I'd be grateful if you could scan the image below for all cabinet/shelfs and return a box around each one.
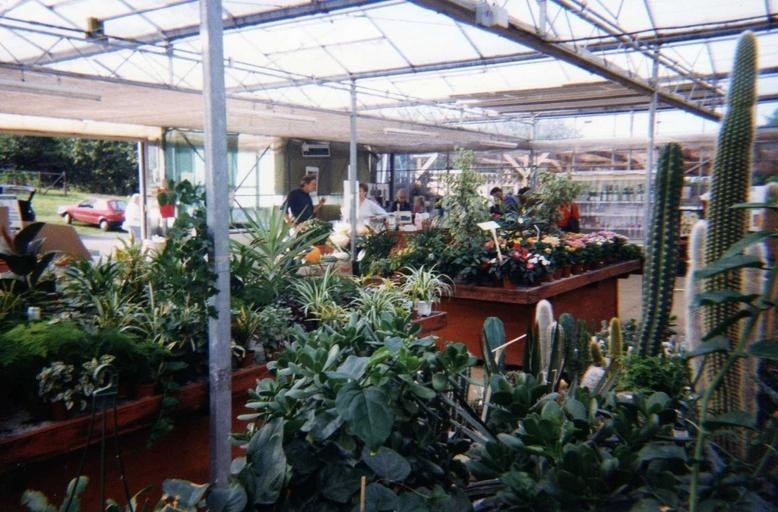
[414,169,710,243]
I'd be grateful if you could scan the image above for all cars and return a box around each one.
[56,196,128,231]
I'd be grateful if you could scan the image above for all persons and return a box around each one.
[0,186,20,257]
[125,190,142,239]
[281,173,328,237]
[345,182,389,230]
[389,183,581,237]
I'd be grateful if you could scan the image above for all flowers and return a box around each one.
[481,229,644,286]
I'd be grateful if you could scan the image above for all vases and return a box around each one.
[503,271,517,289]
